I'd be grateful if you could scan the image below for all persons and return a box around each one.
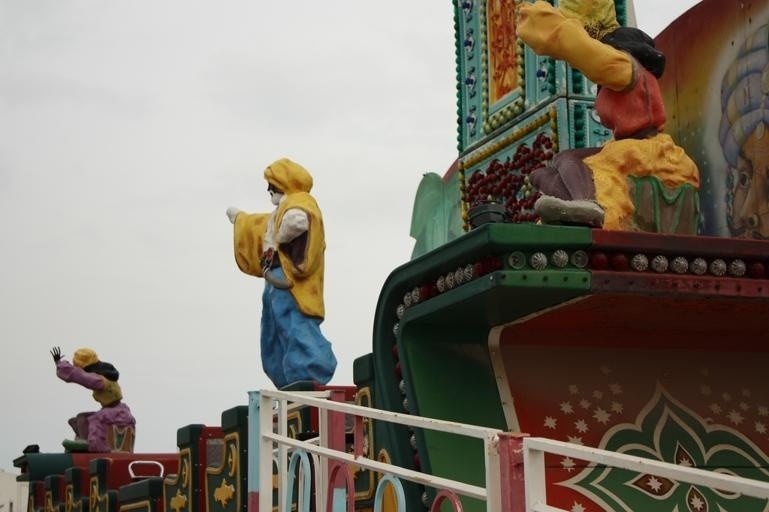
[224,155,336,390]
[510,0,707,238]
[49,346,142,457]
